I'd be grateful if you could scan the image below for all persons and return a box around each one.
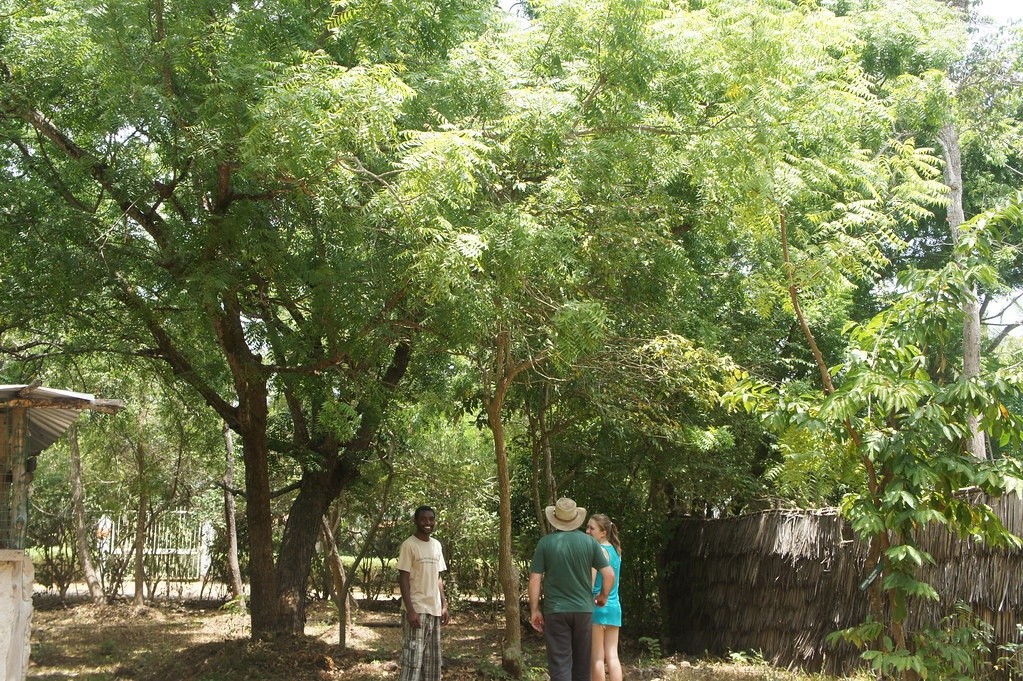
[528,496,614,681]
[397,506,450,681]
[584,514,625,681]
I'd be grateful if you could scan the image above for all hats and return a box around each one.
[545,497,587,531]
